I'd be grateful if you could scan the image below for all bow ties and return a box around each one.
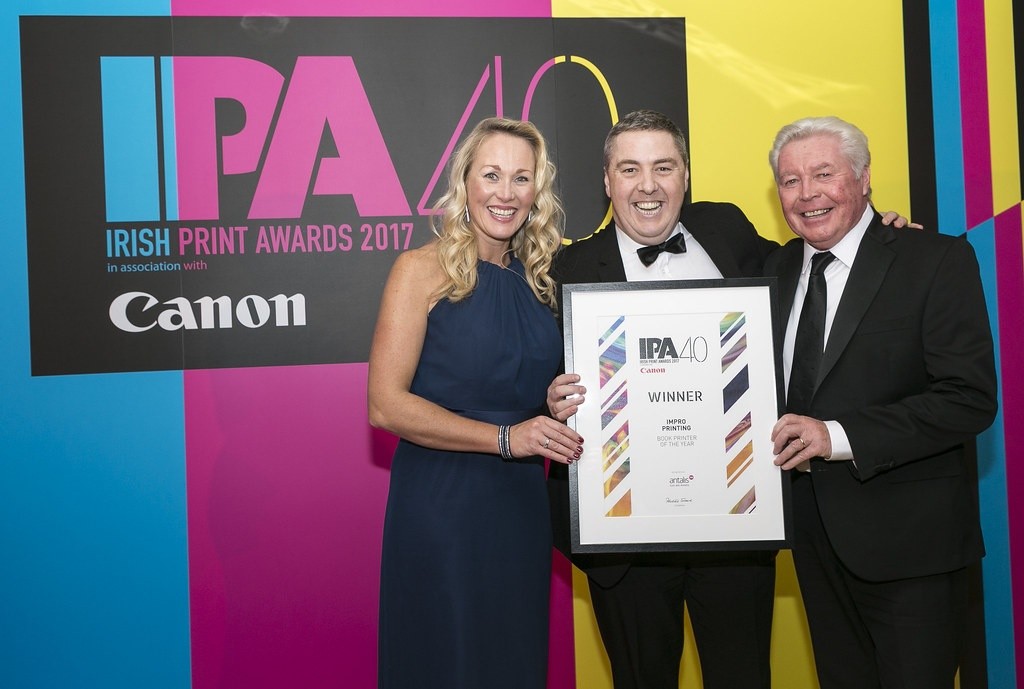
[637,232,687,268]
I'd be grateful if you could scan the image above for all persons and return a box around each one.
[547,109,925,688]
[760,115,999,689]
[368,117,584,688]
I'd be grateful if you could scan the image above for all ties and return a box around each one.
[787,251,835,418]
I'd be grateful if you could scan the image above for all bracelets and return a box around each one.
[498,425,512,459]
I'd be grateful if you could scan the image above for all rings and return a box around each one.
[799,437,806,447]
[544,438,550,448]
